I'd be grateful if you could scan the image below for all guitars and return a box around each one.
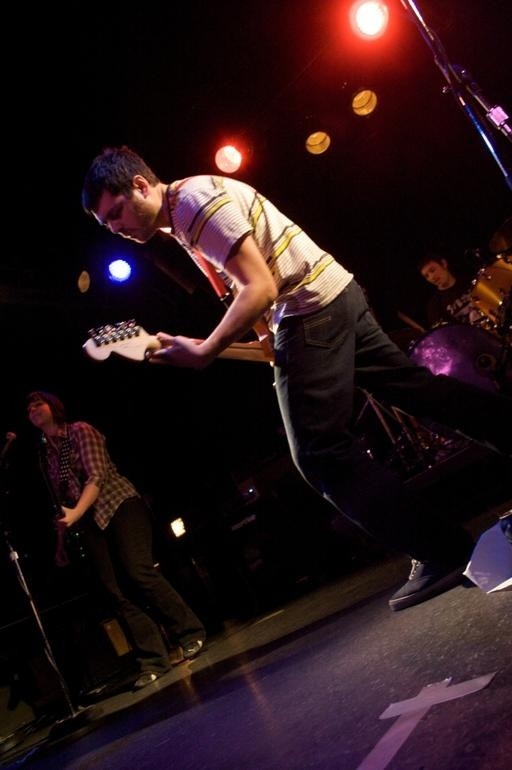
[45,447,81,565]
[84,316,278,374]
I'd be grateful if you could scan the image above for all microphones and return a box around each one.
[0,431,17,457]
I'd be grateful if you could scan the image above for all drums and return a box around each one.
[447,297,505,335]
[407,319,504,397]
[468,255,512,313]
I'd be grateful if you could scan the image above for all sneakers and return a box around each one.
[134,674,157,687]
[183,639,203,659]
[388,558,465,611]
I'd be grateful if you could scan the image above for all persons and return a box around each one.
[418,253,483,324]
[26,391,207,689]
[81,145,512,611]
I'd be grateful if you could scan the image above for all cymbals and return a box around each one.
[488,218,512,252]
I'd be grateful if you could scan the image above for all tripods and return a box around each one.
[354,384,433,477]
